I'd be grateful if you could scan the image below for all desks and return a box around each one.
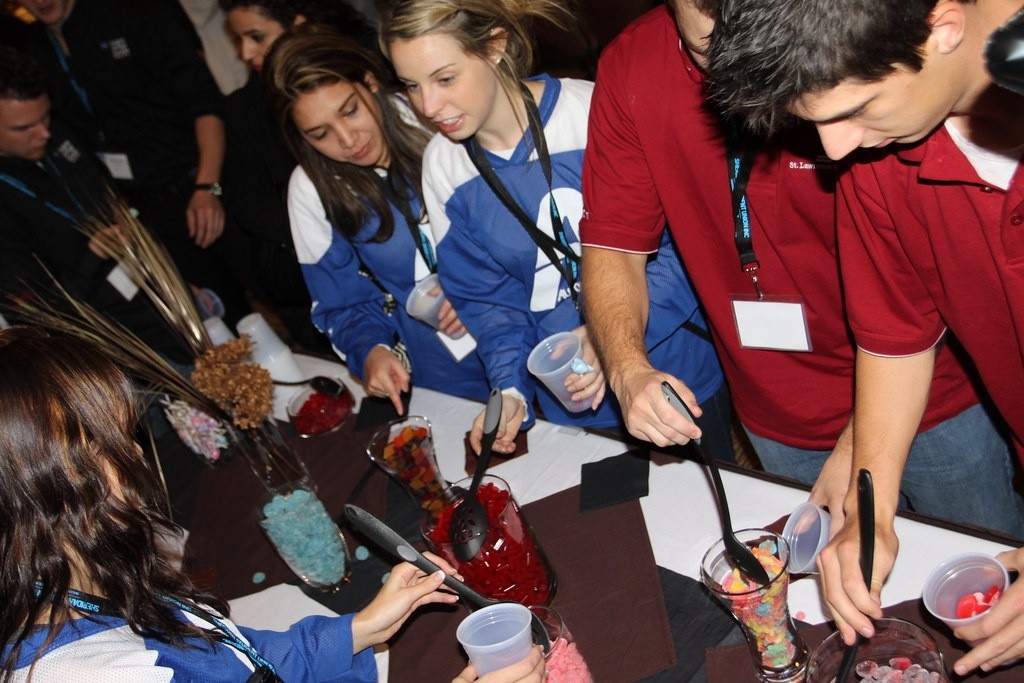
[133,349,1024,683]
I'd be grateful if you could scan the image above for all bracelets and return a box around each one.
[195,183,223,195]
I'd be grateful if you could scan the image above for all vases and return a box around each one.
[226,409,320,500]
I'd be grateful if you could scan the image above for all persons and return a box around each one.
[0,327,547,683]
[377,0,735,463]
[263,23,492,416]
[1,0,402,358]
[578,1,1024,543]
[700,0,1024,676]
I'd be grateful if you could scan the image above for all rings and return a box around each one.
[384,393,389,397]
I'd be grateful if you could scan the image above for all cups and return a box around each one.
[455,602,532,678]
[406,274,466,340]
[287,376,355,440]
[255,481,354,594]
[526,331,596,413]
[203,312,292,374]
[700,528,812,683]
[922,553,1017,665]
[419,474,558,619]
[527,606,593,683]
[782,502,831,575]
[366,414,455,512]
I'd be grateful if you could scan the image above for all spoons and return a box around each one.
[273,376,345,397]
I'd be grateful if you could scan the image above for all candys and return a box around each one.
[718,539,795,668]
[538,637,595,683]
[957,584,1002,619]
[855,657,943,683]
